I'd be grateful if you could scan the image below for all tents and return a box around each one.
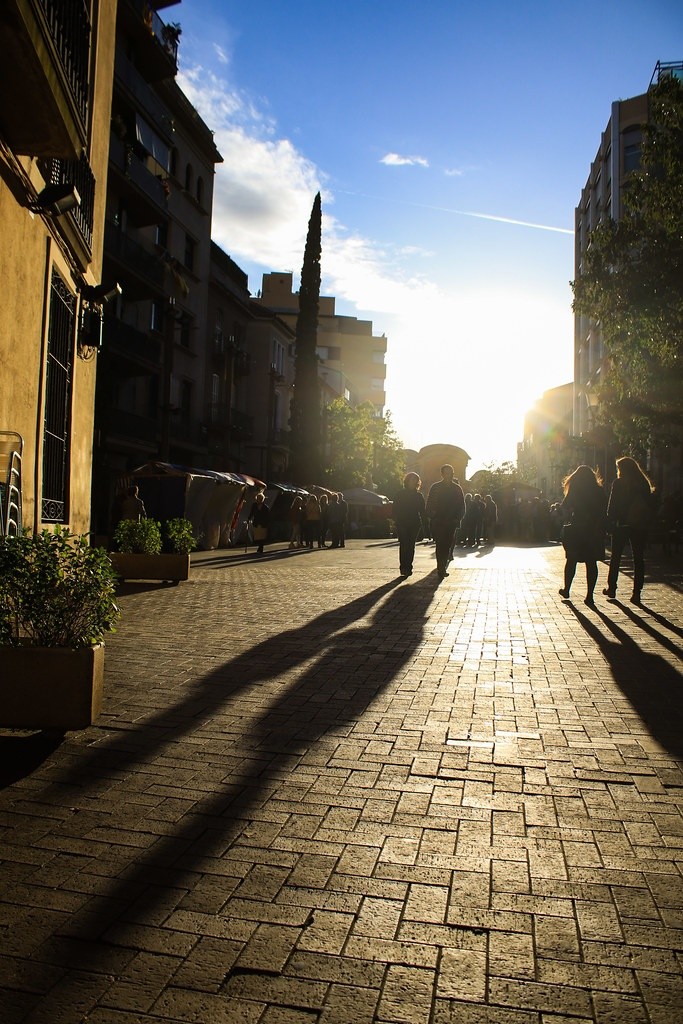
[342,488,389,506]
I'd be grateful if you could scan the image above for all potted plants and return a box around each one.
[160,22,182,53]
[109,516,197,586]
[0,523,130,728]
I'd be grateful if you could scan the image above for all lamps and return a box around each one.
[38,184,81,217]
[94,282,124,305]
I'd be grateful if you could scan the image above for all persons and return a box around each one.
[558,465,605,603]
[453,478,498,547]
[247,493,271,553]
[517,496,566,543]
[392,473,426,576]
[111,486,146,531]
[603,457,654,603]
[659,491,683,556]
[289,492,348,548]
[425,465,466,577]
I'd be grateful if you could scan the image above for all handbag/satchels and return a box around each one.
[286,508,304,522]
[253,525,267,541]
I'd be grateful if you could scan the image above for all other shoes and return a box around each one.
[289,544,296,548]
[584,597,594,607]
[559,589,569,598]
[438,571,449,577]
[630,589,641,604]
[322,544,328,547]
[602,584,617,598]
[318,544,322,548]
